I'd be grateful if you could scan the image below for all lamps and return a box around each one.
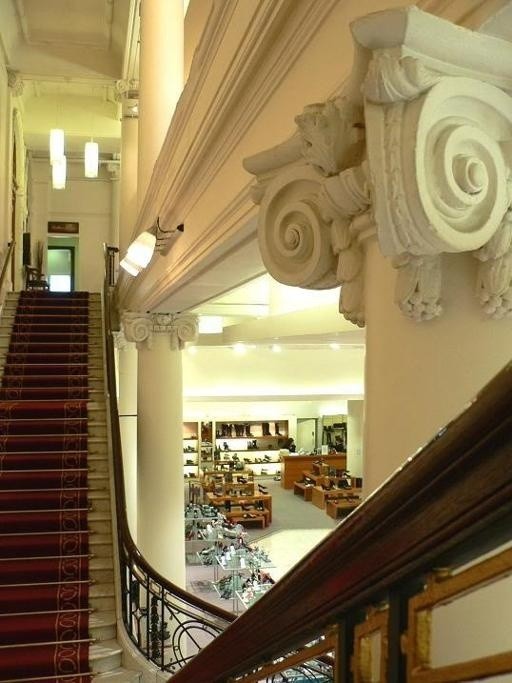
[50,121,100,191]
[118,216,187,277]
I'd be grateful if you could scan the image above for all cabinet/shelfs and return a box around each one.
[181,421,289,477]
[186,507,278,616]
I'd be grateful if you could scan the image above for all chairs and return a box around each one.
[24,263,51,292]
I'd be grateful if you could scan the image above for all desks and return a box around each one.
[293,469,362,519]
[195,467,272,528]
